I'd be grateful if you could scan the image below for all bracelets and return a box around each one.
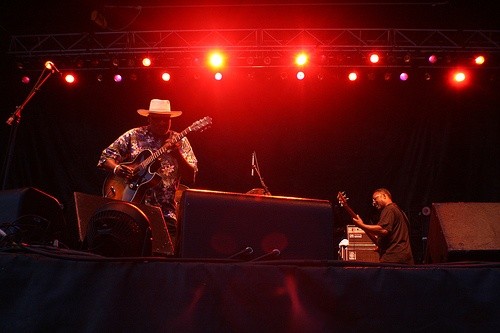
[114,165,119,174]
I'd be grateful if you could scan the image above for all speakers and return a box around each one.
[0,187,63,248]
[63,192,173,258]
[418,202,499,263]
[341,247,381,262]
[173,188,335,262]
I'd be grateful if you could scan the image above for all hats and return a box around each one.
[136,98,183,119]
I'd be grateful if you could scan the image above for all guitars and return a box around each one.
[103,116,213,205]
[337,192,383,255]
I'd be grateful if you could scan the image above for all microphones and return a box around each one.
[226,247,253,260]
[249,249,281,261]
[251,152,255,176]
[49,62,63,78]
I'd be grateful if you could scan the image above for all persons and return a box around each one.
[354,188,414,265]
[97,99,198,256]
